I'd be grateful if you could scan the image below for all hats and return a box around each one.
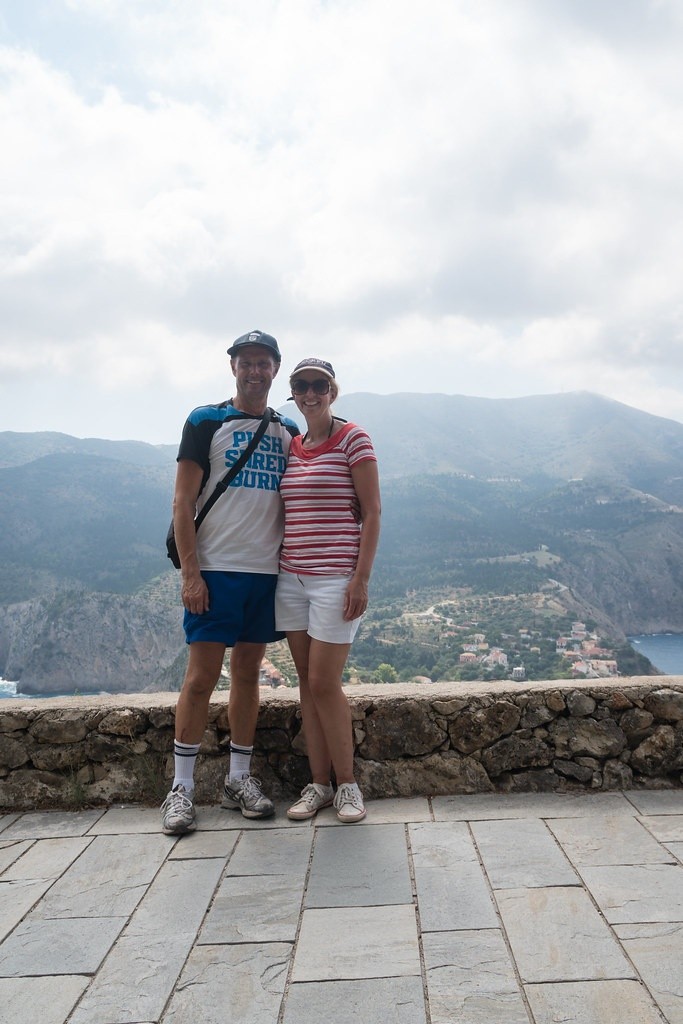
[289,358,335,387]
[227,330,280,362]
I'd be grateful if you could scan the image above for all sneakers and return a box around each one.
[160,783,196,834]
[286,779,337,819]
[332,782,367,823]
[221,773,275,819]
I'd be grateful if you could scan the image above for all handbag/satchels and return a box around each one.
[165,519,181,569]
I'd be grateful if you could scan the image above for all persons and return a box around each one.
[158,330,365,831]
[275,358,383,824]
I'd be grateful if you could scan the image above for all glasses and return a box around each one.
[291,378,331,395]
